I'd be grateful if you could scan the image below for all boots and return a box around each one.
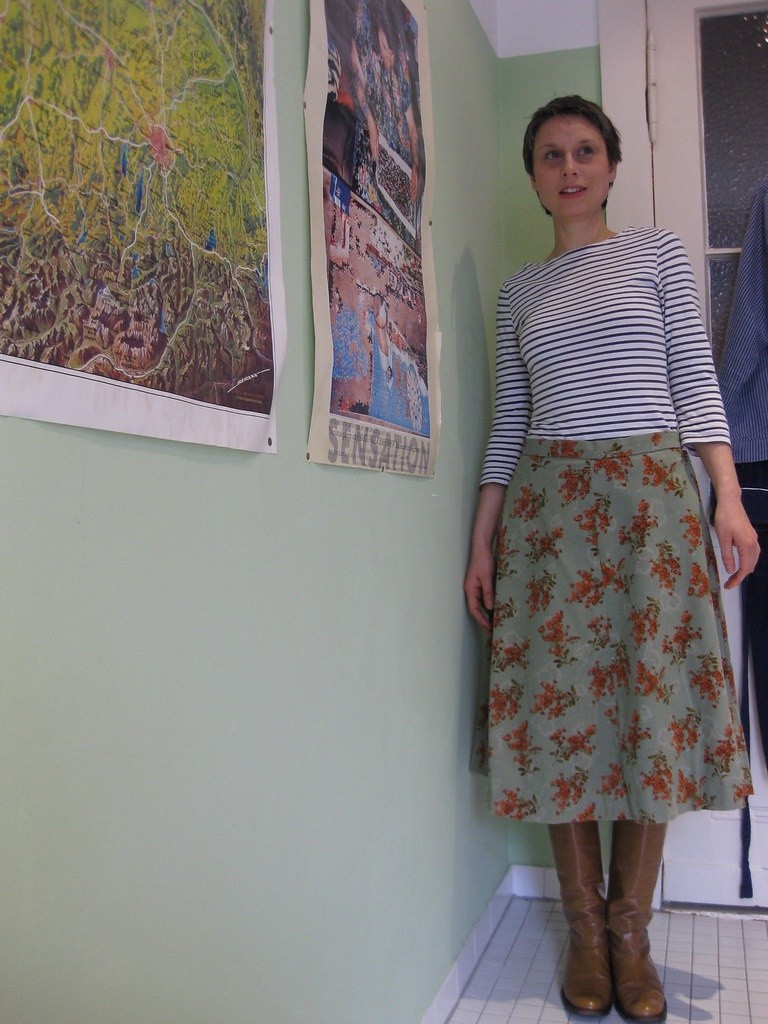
[548,821,668,1023]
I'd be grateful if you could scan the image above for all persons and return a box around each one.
[346,1,421,202]
[464,95,760,1023]
